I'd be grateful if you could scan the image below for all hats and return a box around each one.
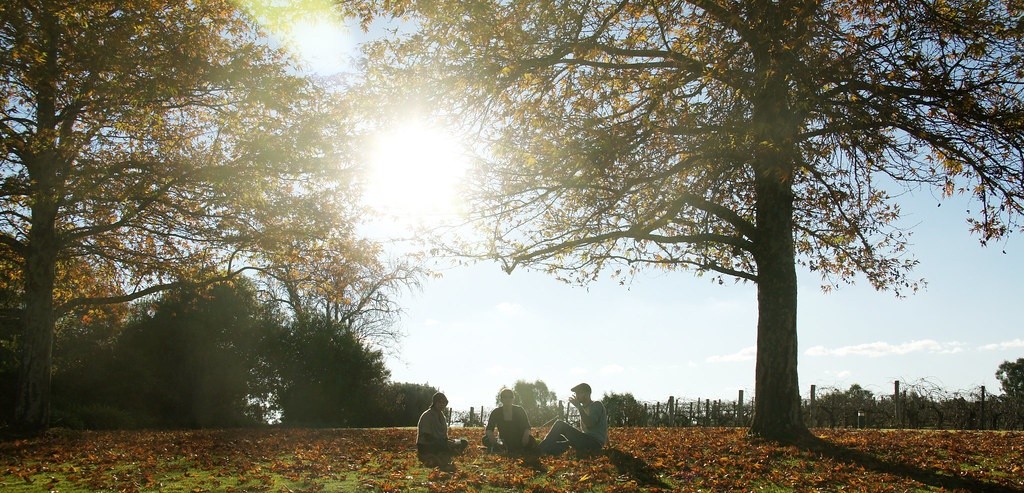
[429,392,448,408]
[571,383,591,394]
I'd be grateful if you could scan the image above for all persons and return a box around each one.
[417,392,468,455]
[482,389,540,450]
[539,383,608,453]
